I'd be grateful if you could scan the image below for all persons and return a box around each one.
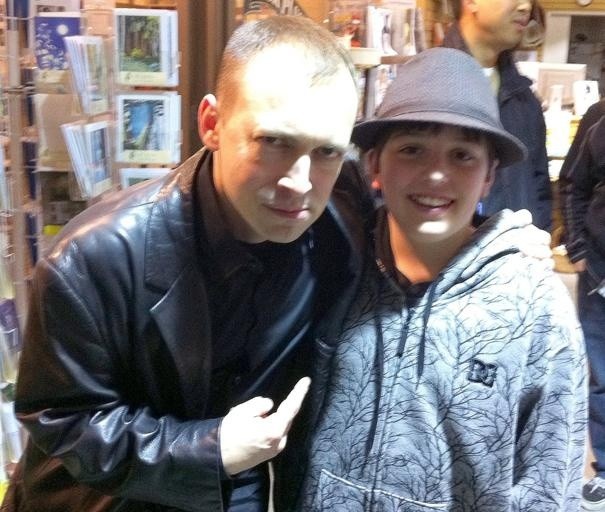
[281,47,591,512]
[558,99,605,511]
[439,0,553,233]
[14,14,555,512]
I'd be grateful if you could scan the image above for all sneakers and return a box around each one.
[580,476,605,511]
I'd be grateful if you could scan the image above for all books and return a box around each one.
[515,61,598,182]
[1,0,180,483]
[365,0,427,118]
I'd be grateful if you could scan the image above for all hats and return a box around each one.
[352,46,528,169]
